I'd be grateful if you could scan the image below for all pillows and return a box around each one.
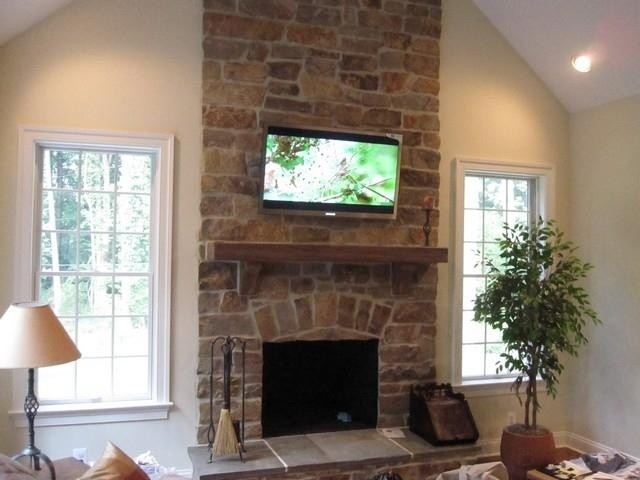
[79,440,152,480]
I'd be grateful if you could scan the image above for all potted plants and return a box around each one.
[472,215,602,480]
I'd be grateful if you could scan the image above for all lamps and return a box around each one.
[1,300,80,480]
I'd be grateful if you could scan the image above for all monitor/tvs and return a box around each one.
[259,120,403,221]
